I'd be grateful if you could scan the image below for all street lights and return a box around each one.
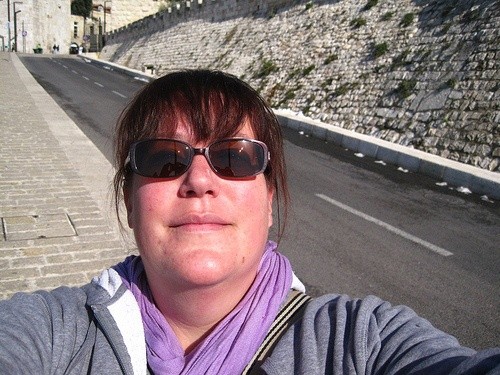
[104,0,112,33]
[13,1,23,52]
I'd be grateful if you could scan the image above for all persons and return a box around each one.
[0,70,500,375]
